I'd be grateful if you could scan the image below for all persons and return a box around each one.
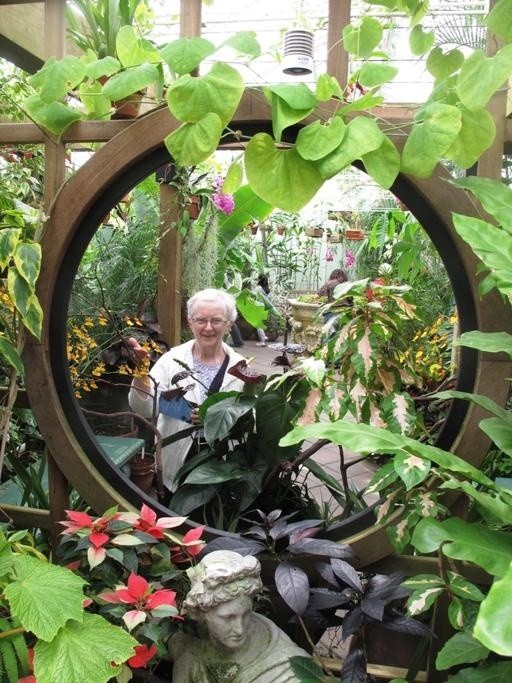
[221,266,247,347]
[160,548,318,683]
[123,287,252,529]
[253,273,271,345]
[313,268,351,371]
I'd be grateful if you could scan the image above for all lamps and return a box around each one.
[282,0,317,77]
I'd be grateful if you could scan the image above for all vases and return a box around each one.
[128,451,156,494]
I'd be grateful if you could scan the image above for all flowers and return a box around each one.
[62,305,162,401]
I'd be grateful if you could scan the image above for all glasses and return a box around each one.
[189,316,229,326]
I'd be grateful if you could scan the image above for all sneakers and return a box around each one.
[255,342,266,347]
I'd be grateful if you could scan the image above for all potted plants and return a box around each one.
[247,211,365,243]
[66,0,156,119]
[168,165,216,220]
